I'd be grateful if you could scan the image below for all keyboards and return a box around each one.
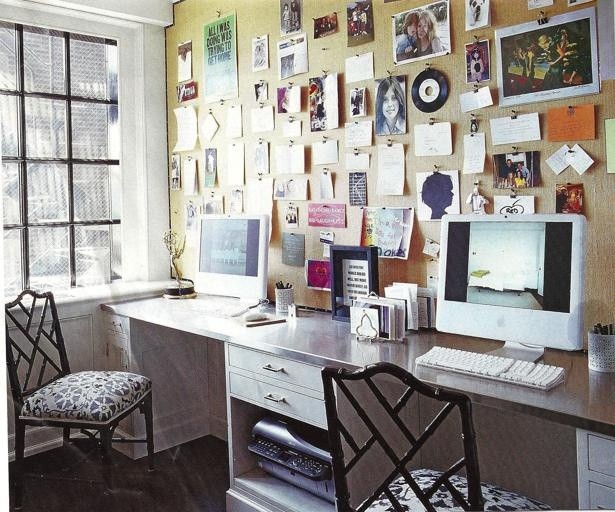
[414,344,566,392]
[180,297,249,317]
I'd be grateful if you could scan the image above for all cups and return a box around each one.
[275,288,294,312]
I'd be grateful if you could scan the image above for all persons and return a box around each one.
[498,158,531,187]
[375,77,407,134]
[505,30,586,89]
[347,5,371,37]
[289,1,300,30]
[281,4,291,26]
[395,12,419,55]
[421,173,453,219]
[556,187,567,212]
[411,12,444,55]
[565,189,581,212]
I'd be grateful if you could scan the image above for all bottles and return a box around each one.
[288,301,296,318]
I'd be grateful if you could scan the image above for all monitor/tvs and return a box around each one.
[436,214,587,364]
[194,214,270,300]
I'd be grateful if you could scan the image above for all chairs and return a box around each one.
[322,361,560,512]
[5,290,158,511]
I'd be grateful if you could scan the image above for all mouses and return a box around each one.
[245,310,268,321]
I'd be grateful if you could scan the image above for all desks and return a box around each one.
[100,287,615,510]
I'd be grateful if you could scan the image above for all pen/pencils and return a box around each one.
[247,319,286,327]
[276,281,292,289]
[594,322,612,335]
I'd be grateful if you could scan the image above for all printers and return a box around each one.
[246,410,335,505]
